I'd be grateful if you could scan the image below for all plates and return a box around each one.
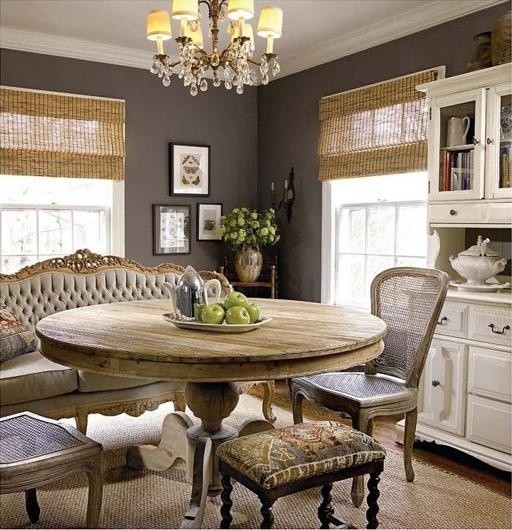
[160,313,273,333]
[449,280,512,293]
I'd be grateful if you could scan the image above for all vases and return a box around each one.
[234,245,262,283]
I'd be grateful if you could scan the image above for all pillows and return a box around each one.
[0,306,36,362]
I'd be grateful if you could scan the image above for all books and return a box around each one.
[500,146,511,188]
[439,151,474,190]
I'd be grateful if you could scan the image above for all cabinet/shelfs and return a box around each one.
[416,63,512,236]
[395,284,468,454]
[469,290,512,477]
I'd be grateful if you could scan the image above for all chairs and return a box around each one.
[292,267,449,507]
[0,409,103,530]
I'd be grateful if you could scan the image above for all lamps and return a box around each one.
[272,166,297,223]
[147,0,283,96]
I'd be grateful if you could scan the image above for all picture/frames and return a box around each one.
[196,203,223,241]
[152,203,191,255]
[169,142,211,198]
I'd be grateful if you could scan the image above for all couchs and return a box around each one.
[0,249,278,432]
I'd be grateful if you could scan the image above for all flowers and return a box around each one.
[218,208,281,247]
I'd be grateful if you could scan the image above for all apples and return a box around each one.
[226,305,250,325]
[224,291,249,310]
[202,303,226,324]
[247,304,260,324]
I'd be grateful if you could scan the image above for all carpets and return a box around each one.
[0,395,512,529]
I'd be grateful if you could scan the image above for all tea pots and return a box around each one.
[163,266,221,322]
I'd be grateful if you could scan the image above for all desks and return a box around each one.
[34,298,387,529]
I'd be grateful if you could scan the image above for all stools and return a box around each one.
[215,420,386,529]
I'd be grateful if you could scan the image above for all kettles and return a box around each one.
[446,116,470,147]
